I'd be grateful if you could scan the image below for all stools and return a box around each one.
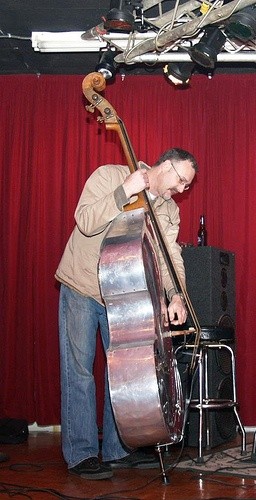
[185,326,247,465]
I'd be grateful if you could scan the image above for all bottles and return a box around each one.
[198,215,207,246]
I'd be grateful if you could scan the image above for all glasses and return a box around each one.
[162,160,190,190]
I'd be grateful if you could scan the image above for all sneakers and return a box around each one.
[68,457,113,480]
[102,449,161,469]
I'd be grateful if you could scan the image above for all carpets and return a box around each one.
[175,443,256,479]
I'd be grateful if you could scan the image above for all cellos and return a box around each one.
[81,72,203,455]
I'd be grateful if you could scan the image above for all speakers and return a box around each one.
[169,245,236,345]
[173,345,238,451]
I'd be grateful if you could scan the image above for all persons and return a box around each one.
[54,146,200,480]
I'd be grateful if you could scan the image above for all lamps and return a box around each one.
[96,42,120,80]
[104,0,143,32]
[189,27,226,69]
[163,49,193,89]
[225,5,256,45]
